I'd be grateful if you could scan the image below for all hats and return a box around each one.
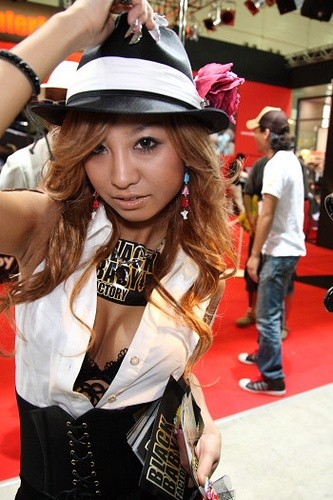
[30,10,230,137]
[246,106,281,128]
[259,111,290,135]
[39,60,78,89]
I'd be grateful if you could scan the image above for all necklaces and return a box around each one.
[114,237,166,265]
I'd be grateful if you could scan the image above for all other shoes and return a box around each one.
[236,315,255,326]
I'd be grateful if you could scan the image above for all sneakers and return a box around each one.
[240,377,287,395]
[239,352,258,365]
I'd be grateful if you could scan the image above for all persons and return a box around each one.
[0,59,80,193]
[235,107,306,396]
[0,0,234,500]
[229,153,246,188]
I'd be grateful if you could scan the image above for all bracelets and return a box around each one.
[0,50,40,95]
